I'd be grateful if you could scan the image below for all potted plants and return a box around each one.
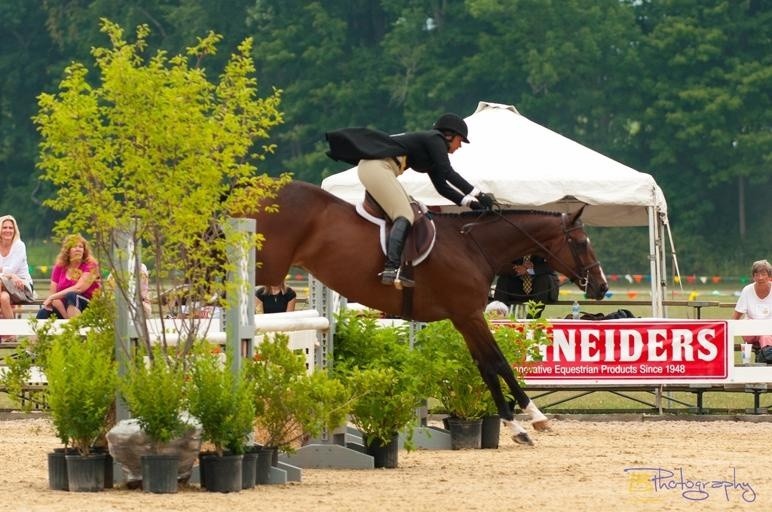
[49,367,75,492]
[188,322,244,492]
[225,357,260,489]
[1,292,114,454]
[198,399,230,491]
[60,338,112,493]
[118,338,189,495]
[254,362,395,483]
[338,310,401,470]
[479,330,526,451]
[254,335,313,467]
[416,308,482,451]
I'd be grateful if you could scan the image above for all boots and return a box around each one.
[380,216,416,289]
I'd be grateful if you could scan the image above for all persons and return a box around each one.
[35,233,102,319]
[494,253,559,304]
[105,262,151,319]
[731,259,772,364]
[167,300,221,319]
[0,214,33,319]
[357,113,494,289]
[485,300,508,320]
[255,277,296,314]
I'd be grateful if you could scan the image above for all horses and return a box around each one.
[142,175,609,448]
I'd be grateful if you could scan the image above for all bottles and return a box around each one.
[572,299,581,319]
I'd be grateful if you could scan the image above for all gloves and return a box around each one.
[475,191,493,210]
[469,200,479,211]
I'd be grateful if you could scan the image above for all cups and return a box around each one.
[741,344,752,365]
[515,303,529,321]
[510,305,515,316]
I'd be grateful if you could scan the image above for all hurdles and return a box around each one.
[192,219,375,485]
[112,217,321,489]
[399,319,451,450]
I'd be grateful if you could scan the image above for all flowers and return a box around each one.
[483,301,554,450]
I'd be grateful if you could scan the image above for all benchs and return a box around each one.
[490,299,771,415]
[1,311,312,390]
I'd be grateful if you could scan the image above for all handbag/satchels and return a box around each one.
[0,273,35,304]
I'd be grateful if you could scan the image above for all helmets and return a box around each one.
[432,114,471,144]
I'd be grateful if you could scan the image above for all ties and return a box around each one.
[522,256,533,295]
